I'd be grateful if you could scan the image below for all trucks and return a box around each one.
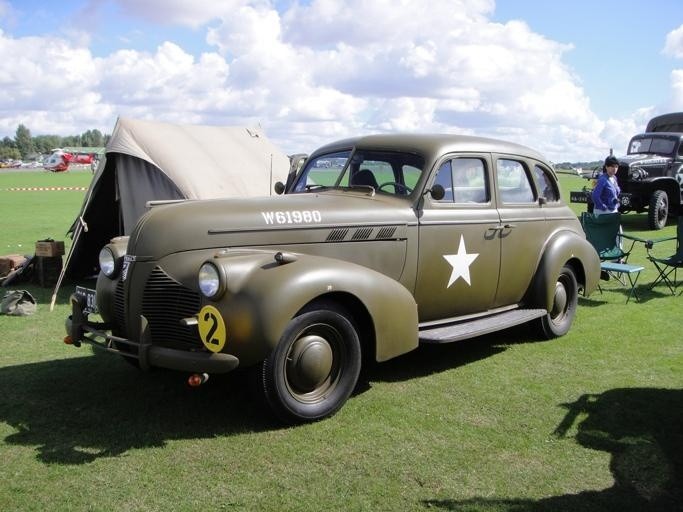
[565,112,683,228]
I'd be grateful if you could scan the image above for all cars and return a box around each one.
[0,154,42,170]
[53,130,601,429]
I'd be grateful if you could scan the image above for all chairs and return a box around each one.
[349,169,380,191]
[581,211,683,305]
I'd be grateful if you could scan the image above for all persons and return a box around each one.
[590,155,622,280]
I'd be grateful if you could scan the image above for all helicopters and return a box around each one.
[29,141,98,175]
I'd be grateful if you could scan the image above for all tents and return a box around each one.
[50,118,316,312]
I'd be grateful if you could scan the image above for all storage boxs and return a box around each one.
[0,240,64,288]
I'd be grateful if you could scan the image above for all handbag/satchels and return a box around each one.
[618,193,632,207]
[0,290,36,316]
